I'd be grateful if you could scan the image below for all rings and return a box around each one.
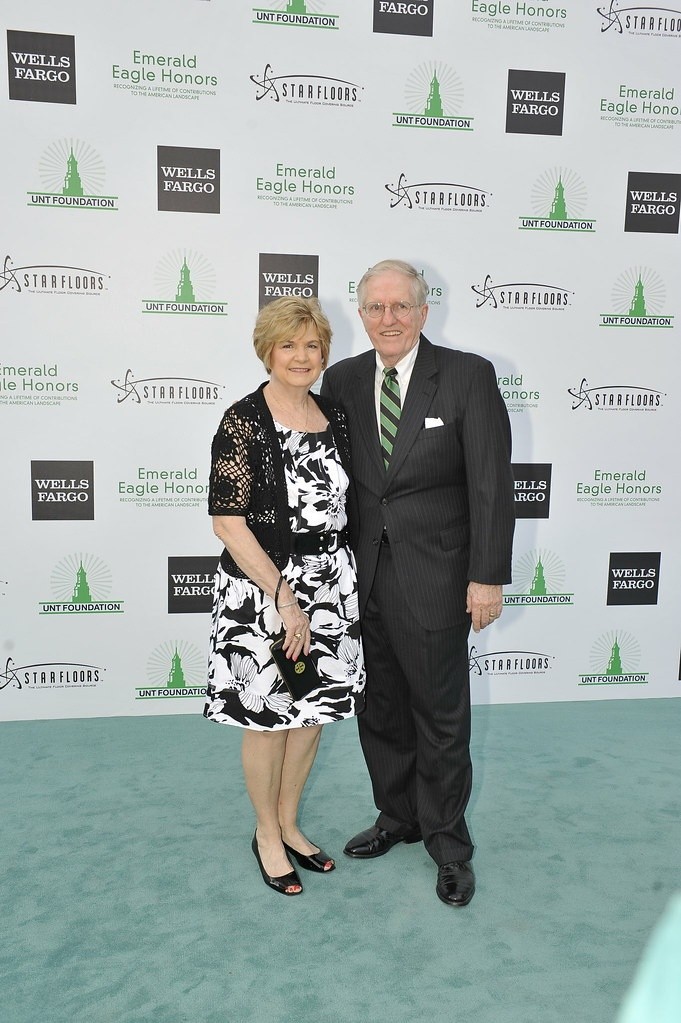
[489,613,497,616]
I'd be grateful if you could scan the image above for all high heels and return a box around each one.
[252,827,302,896]
[282,833,336,873]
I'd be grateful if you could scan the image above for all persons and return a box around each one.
[204,295,368,897]
[317,258,518,909]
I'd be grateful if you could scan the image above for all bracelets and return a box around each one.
[277,597,299,608]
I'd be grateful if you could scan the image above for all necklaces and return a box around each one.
[267,384,309,438]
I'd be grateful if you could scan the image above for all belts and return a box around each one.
[256,526,349,556]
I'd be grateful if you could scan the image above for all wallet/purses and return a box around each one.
[270,633,322,702]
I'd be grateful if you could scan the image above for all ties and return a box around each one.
[381,368,401,470]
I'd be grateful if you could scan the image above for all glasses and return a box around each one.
[362,301,416,318]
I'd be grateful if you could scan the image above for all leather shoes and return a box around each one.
[343,824,423,859]
[436,860,476,907]
